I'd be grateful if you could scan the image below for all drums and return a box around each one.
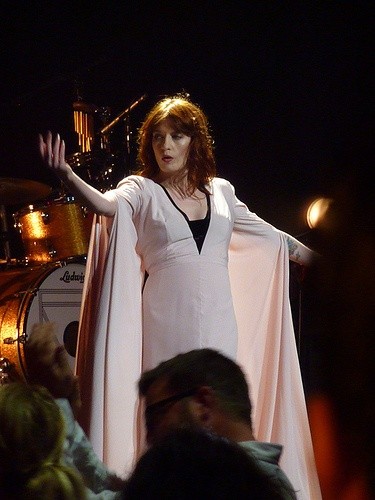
[14,192,93,264]
[0,254,87,399]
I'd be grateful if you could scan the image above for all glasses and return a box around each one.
[145,393,194,432]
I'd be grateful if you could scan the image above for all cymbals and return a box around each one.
[0,176,52,206]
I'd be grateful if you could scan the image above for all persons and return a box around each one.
[37,92,323,500]
[0,320,298,500]
